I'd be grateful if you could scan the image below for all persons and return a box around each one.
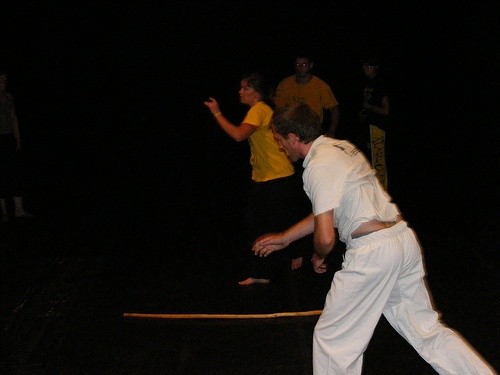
[204,72,304,286]
[253,101,500,375]
[274,55,339,214]
[361,56,390,193]
[0,73,35,223]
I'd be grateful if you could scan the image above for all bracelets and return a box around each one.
[213,111,221,117]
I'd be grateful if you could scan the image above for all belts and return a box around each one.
[349,212,404,239]
